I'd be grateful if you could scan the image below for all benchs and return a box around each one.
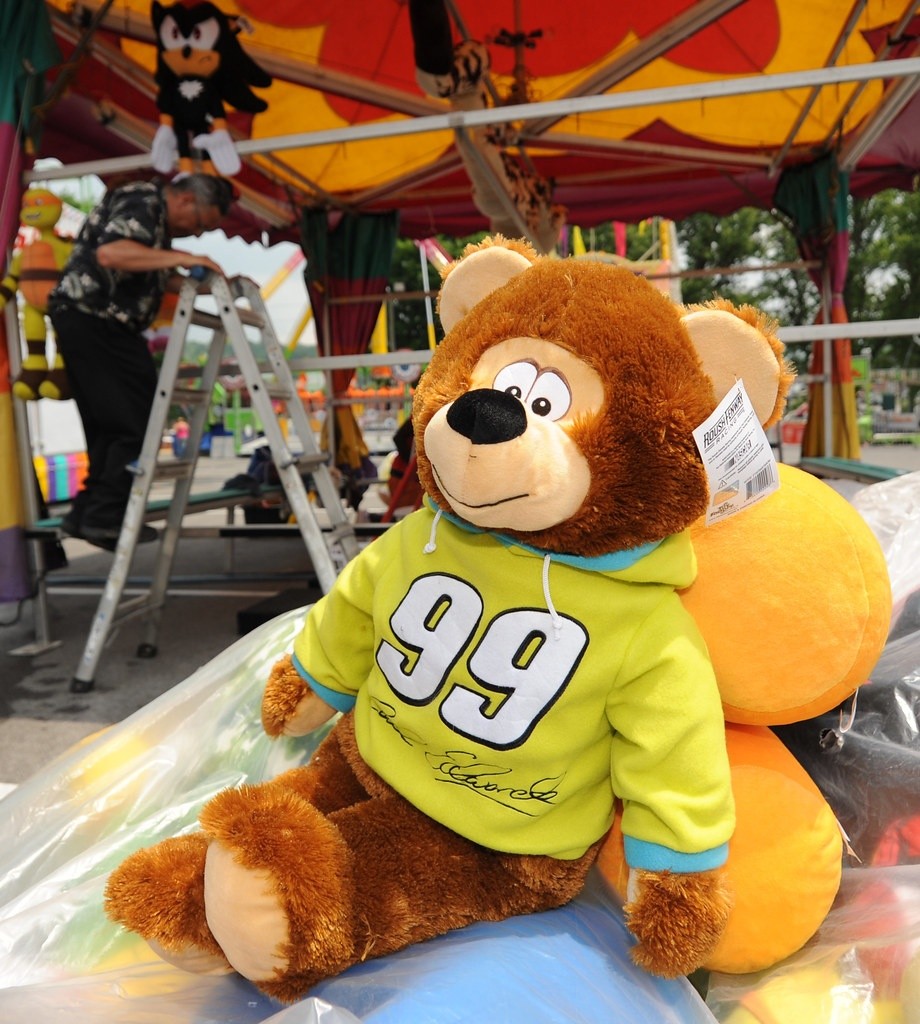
[33,474,348,542]
[799,453,908,483]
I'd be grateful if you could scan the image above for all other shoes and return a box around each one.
[62,510,158,547]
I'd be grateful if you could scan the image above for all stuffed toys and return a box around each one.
[149,0,272,177]
[105,234,794,999]
[598,465,890,975]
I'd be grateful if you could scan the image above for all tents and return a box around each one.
[0,0,919,643]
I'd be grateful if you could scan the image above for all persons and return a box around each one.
[171,416,189,457]
[49,174,238,552]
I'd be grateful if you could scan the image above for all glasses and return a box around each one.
[193,197,205,232]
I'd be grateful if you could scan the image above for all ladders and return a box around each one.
[71,268,364,700]
